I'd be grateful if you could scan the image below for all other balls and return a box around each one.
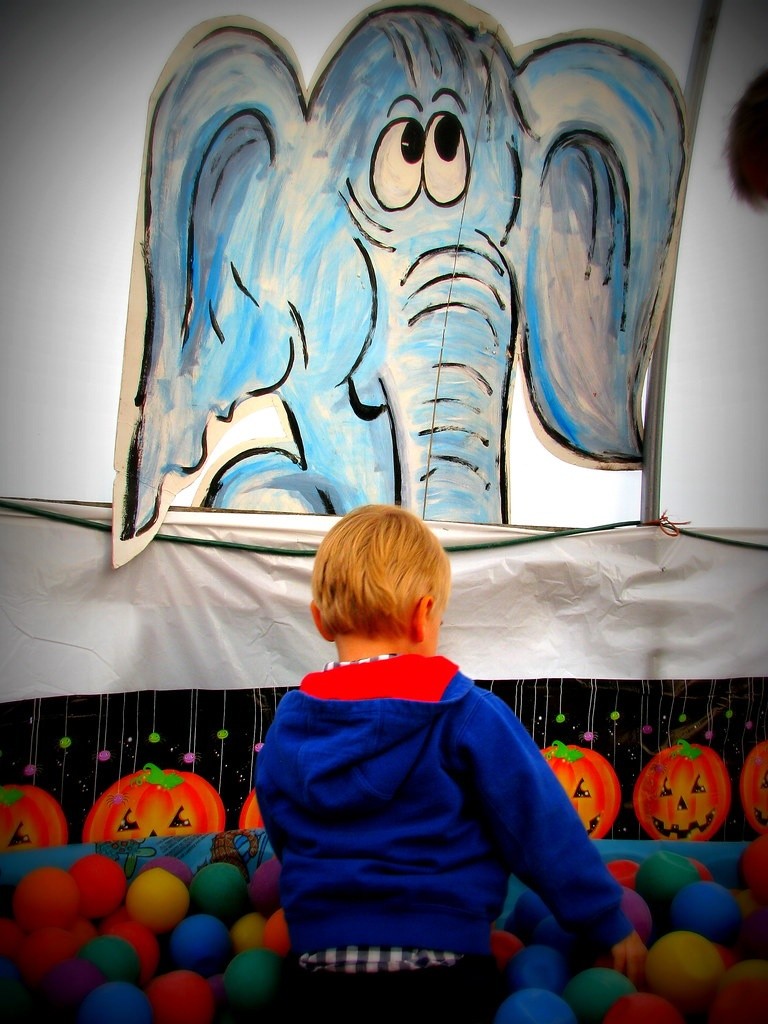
[1,836,768,1023]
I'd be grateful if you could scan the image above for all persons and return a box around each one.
[252,506,649,1024]
